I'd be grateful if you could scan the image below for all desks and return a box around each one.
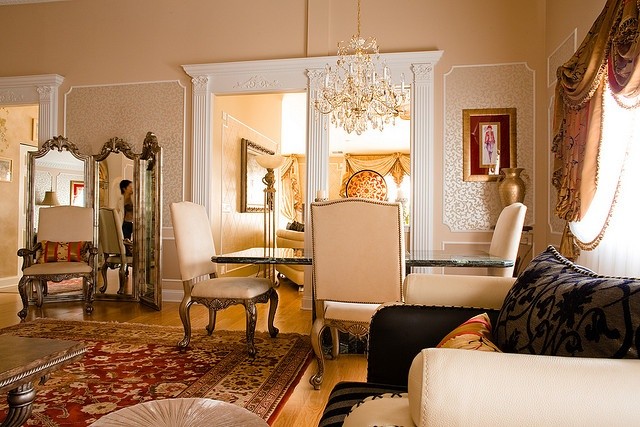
[0,335,88,426]
[211,247,513,356]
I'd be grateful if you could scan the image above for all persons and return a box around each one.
[484,125,495,164]
[119,179,134,257]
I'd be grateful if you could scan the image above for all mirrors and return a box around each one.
[26,132,162,314]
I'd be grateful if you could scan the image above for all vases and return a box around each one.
[499,168,526,207]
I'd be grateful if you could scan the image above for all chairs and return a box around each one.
[16,206,97,322]
[489,204,526,277]
[309,200,406,390]
[99,207,134,295]
[170,202,279,355]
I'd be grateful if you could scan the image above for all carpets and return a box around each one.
[1,318,315,427]
[0,278,84,294]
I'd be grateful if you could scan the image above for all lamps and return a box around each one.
[313,1,406,137]
[36,191,59,207]
[256,154,284,286]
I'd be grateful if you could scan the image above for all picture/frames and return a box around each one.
[70,179,85,208]
[0,157,13,182]
[463,108,518,183]
[241,138,276,214]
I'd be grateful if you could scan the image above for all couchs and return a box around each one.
[317,272,639,426]
[277,222,305,292]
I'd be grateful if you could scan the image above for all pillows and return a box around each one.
[290,221,298,230]
[296,222,305,231]
[438,313,505,354]
[286,222,291,230]
[492,245,640,359]
[37,241,89,264]
